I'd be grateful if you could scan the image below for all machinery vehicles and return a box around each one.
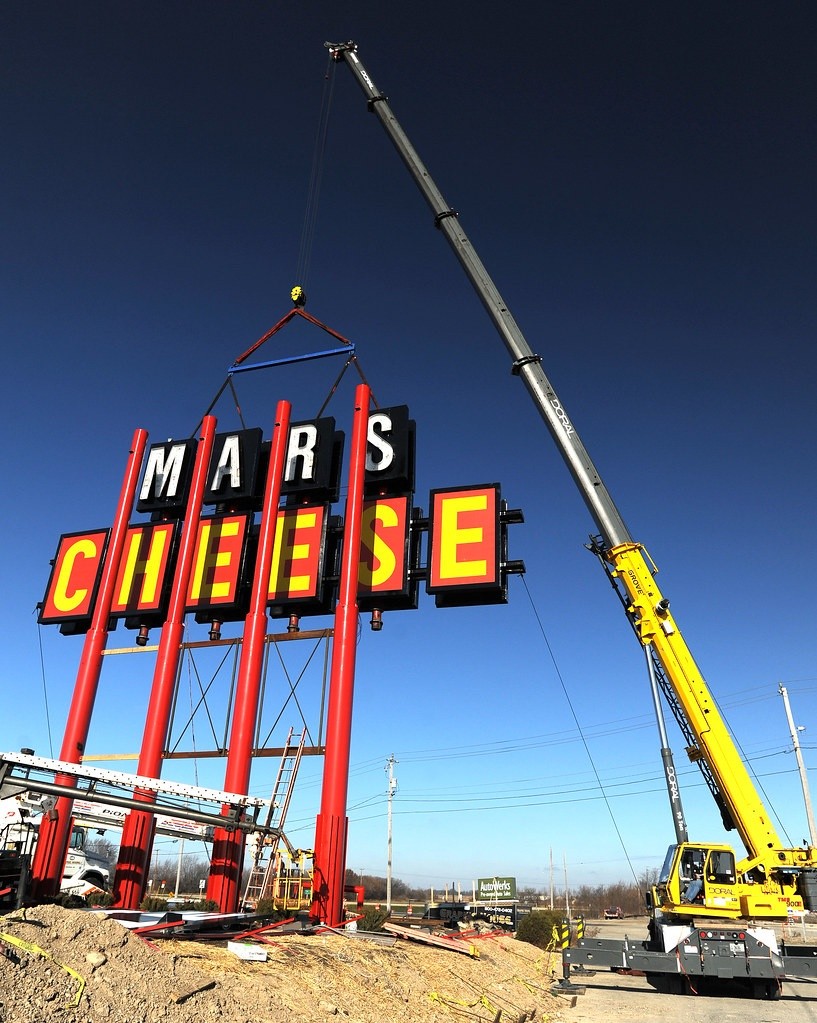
[325,37,817,920]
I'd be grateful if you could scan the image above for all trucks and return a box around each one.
[0,797,110,900]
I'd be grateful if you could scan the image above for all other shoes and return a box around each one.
[681,898,689,904]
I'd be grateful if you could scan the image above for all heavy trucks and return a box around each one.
[644,885,782,1001]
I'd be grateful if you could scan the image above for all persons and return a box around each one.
[680,855,709,903]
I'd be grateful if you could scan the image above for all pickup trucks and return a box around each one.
[603,906,623,919]
